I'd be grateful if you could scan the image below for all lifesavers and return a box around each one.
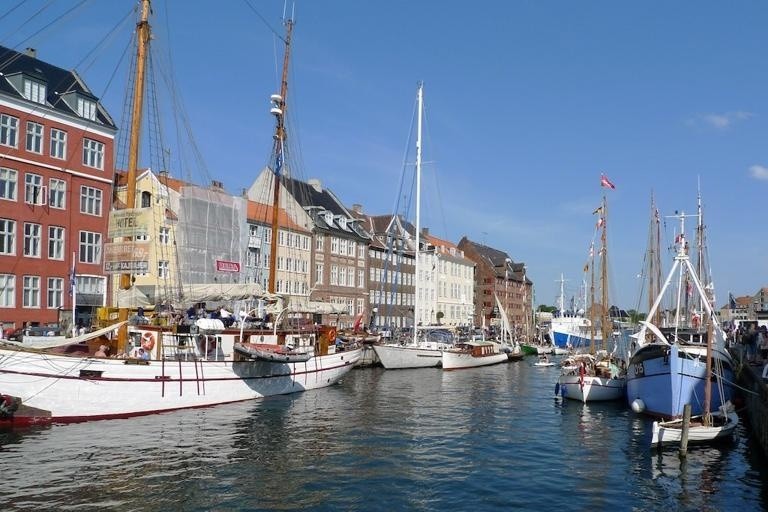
[199,335,216,355]
[329,331,336,341]
[141,332,154,350]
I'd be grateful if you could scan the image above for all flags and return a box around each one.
[601,175,616,191]
[729,292,737,309]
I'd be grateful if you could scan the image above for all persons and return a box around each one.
[95,345,107,357]
[134,348,149,360]
[725,324,768,390]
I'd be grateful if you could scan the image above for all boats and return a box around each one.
[648,407,739,449]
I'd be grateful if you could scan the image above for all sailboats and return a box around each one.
[0,0,361,428]
